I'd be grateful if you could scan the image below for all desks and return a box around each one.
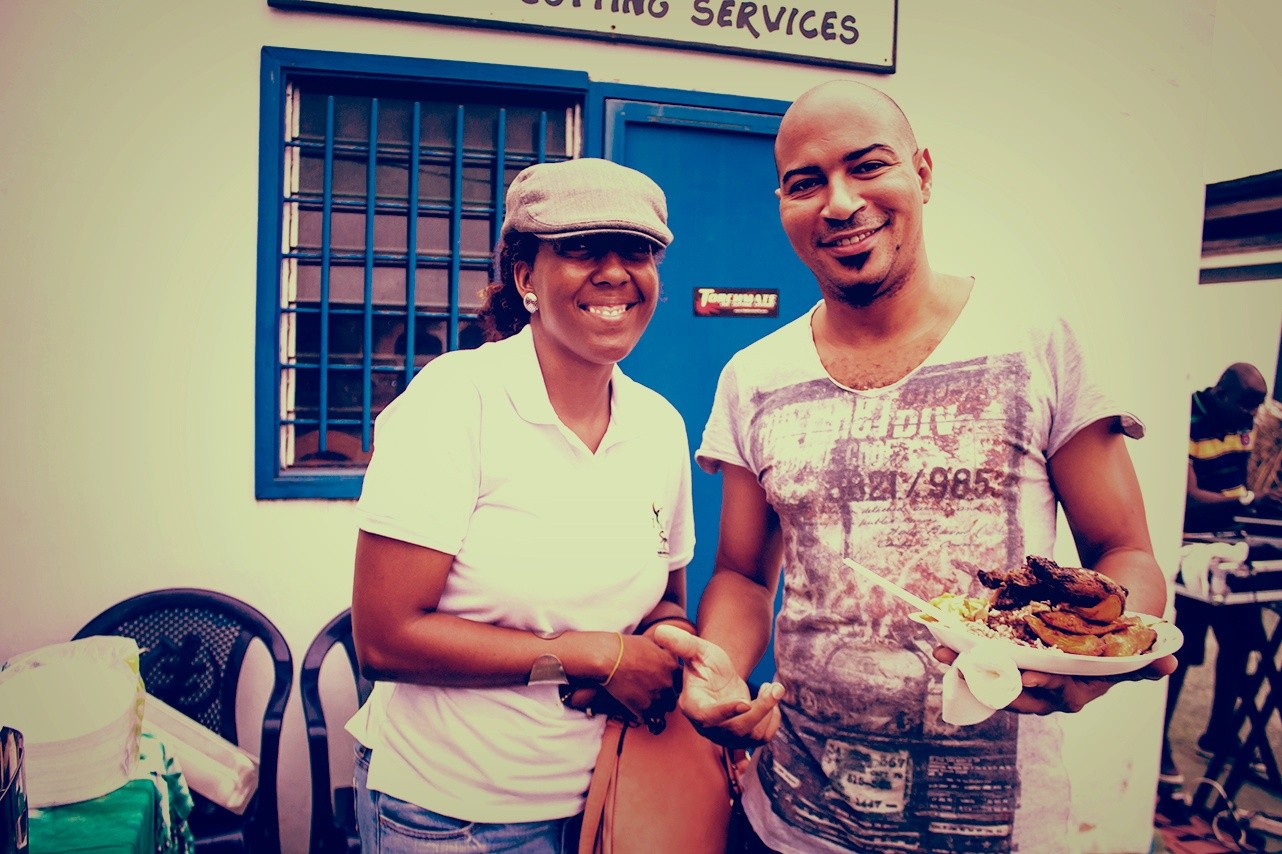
[1174,576,1282,833]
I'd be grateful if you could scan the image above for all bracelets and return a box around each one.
[523,630,569,687]
[600,630,624,686]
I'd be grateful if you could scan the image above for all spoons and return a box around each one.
[843,558,971,634]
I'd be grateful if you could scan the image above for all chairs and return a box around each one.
[299,608,382,853]
[66,584,294,853]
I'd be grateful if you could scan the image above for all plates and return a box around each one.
[908,606,1183,676]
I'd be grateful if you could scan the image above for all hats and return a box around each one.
[500,157,673,252]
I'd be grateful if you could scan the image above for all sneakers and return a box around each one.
[1161,749,1182,784]
[1196,734,1268,772]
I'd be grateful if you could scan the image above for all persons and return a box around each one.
[650,79,1174,852]
[1158,361,1269,782]
[345,151,699,854]
